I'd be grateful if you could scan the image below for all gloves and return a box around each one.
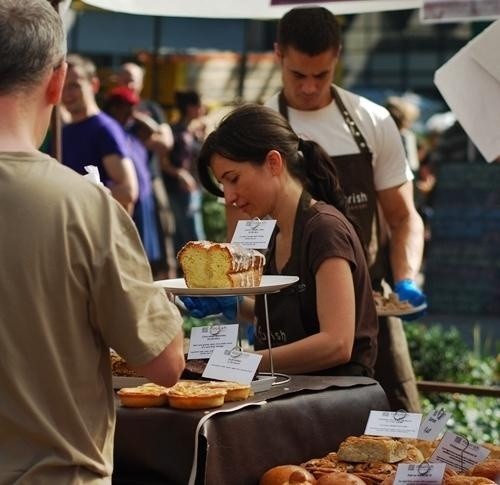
[392,279,429,322]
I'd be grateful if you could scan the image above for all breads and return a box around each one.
[260,425,500,484]
[173,238,264,290]
[114,377,250,411]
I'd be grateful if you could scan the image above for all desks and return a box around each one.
[112,374,392,484]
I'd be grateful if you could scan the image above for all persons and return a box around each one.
[385,97,421,171]
[48,54,209,281]
[0,0,186,485]
[198,103,379,376]
[226,6,424,413]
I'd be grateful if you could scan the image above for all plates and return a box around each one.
[154,273,296,296]
[380,302,425,316]
